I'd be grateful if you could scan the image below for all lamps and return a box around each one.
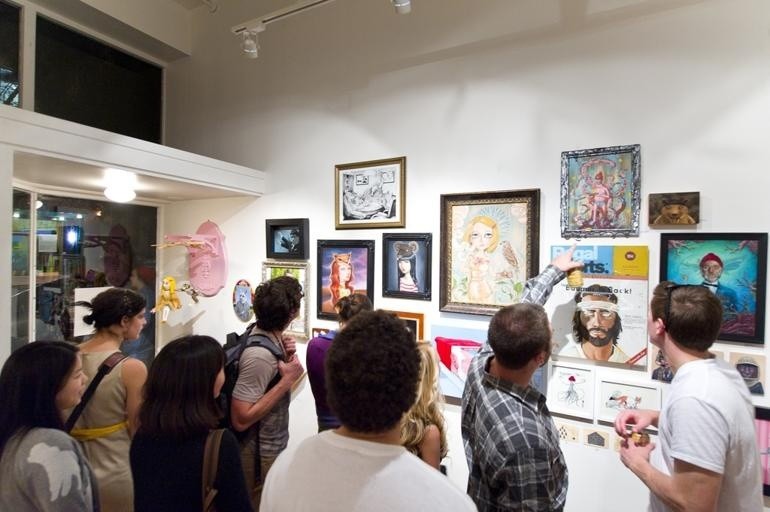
[235,1,411,58]
[103,187,136,204]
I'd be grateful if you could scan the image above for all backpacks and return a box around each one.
[217,333,285,428]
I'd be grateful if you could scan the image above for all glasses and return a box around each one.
[665,284,708,330]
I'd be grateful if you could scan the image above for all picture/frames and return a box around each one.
[392,310,424,341]
[261,261,311,342]
[597,377,661,433]
[264,217,310,261]
[334,155,406,229]
[545,360,594,422]
[381,232,433,301]
[438,187,540,318]
[316,238,375,322]
[752,406,770,496]
[558,143,640,238]
[658,232,765,345]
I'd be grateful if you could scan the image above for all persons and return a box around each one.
[61,286,149,511]
[127,335,254,511]
[616,280,763,511]
[306,292,377,433]
[277,229,300,253]
[121,264,156,369]
[256,308,479,510]
[396,340,451,471]
[220,276,306,510]
[149,275,182,325]
[460,242,585,511]
[0,339,98,511]
